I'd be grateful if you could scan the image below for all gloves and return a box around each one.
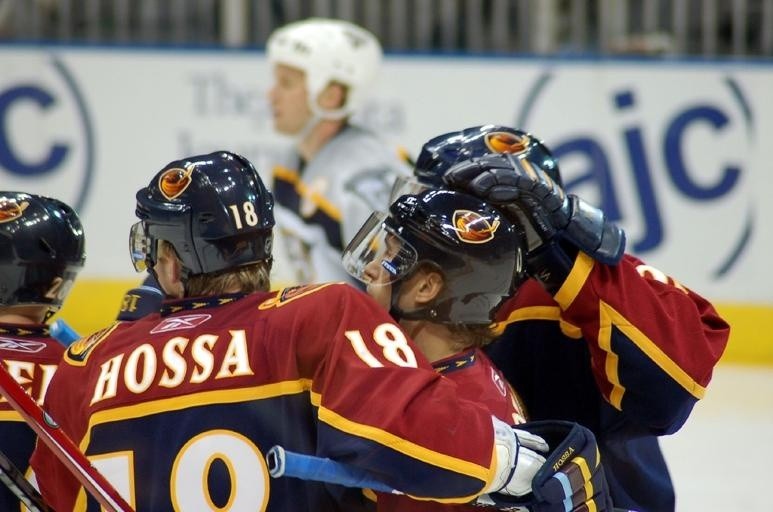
[441,151,629,268]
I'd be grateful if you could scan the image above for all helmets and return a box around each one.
[414,121,566,191]
[1,190,87,310]
[127,148,277,274]
[383,186,518,322]
[268,14,383,127]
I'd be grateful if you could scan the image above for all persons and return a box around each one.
[237,18,414,289]
[0,192,102,511]
[342,193,533,512]
[390,125,731,512]
[30,152,615,511]
